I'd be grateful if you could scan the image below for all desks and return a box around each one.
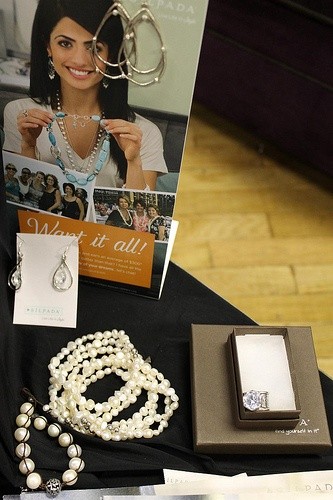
[0,246,333,498]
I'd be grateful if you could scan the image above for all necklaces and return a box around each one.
[149,216,158,226]
[56,90,106,174]
[47,112,110,185]
[116,205,133,226]
[135,211,146,228]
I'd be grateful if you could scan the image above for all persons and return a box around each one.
[56,183,84,221]
[132,198,150,232]
[25,171,46,208]
[105,195,134,230]
[16,168,31,201]
[28,173,36,184]
[75,188,88,220]
[4,162,20,203]
[2,0,169,191]
[38,174,61,215]
[146,204,167,242]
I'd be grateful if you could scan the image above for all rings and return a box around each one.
[23,110,29,117]
